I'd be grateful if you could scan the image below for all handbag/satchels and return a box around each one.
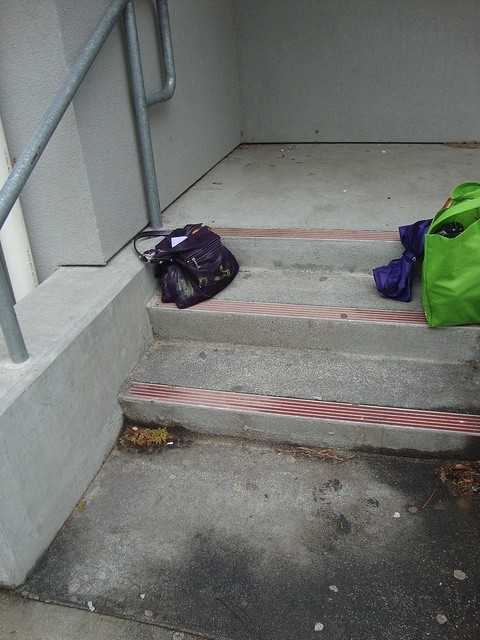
[421,181,480,328]
[134,224,239,310]
